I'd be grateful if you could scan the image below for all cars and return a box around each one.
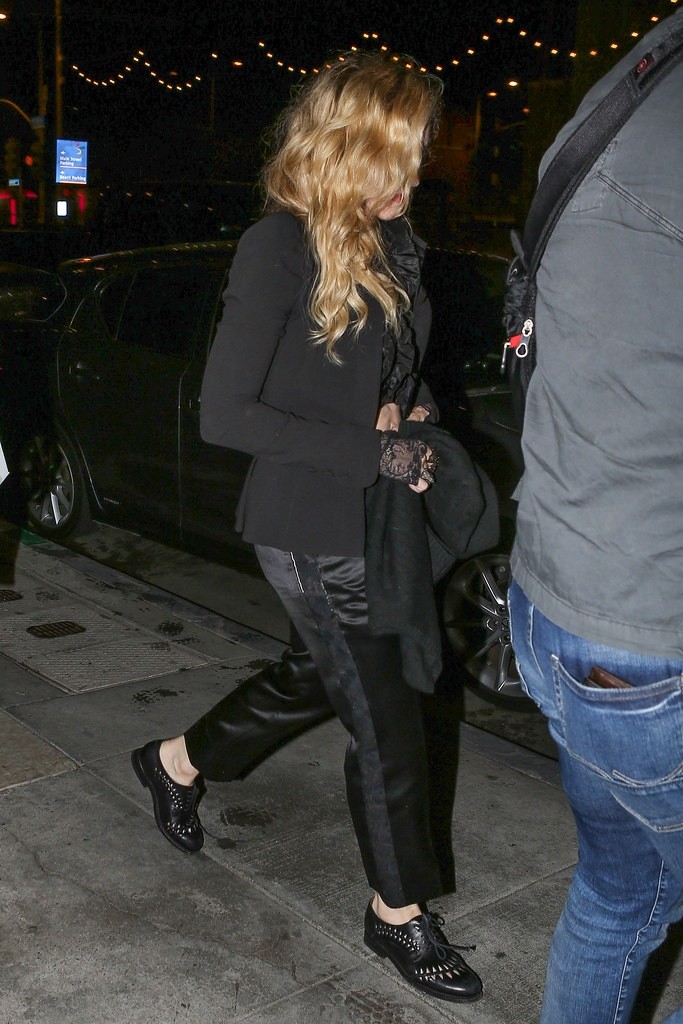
[409,179,466,229]
[480,123,527,163]
[21,239,549,713]
[99,178,262,240]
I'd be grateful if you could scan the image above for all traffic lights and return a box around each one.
[22,150,39,166]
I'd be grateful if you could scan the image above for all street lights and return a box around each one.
[210,61,245,140]
[473,88,496,151]
[0,10,46,226]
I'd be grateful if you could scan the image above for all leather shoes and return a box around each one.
[132,741,204,853]
[363,895,483,1004]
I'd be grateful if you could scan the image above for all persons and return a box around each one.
[130,44,486,1004]
[505,0,683,1024]
